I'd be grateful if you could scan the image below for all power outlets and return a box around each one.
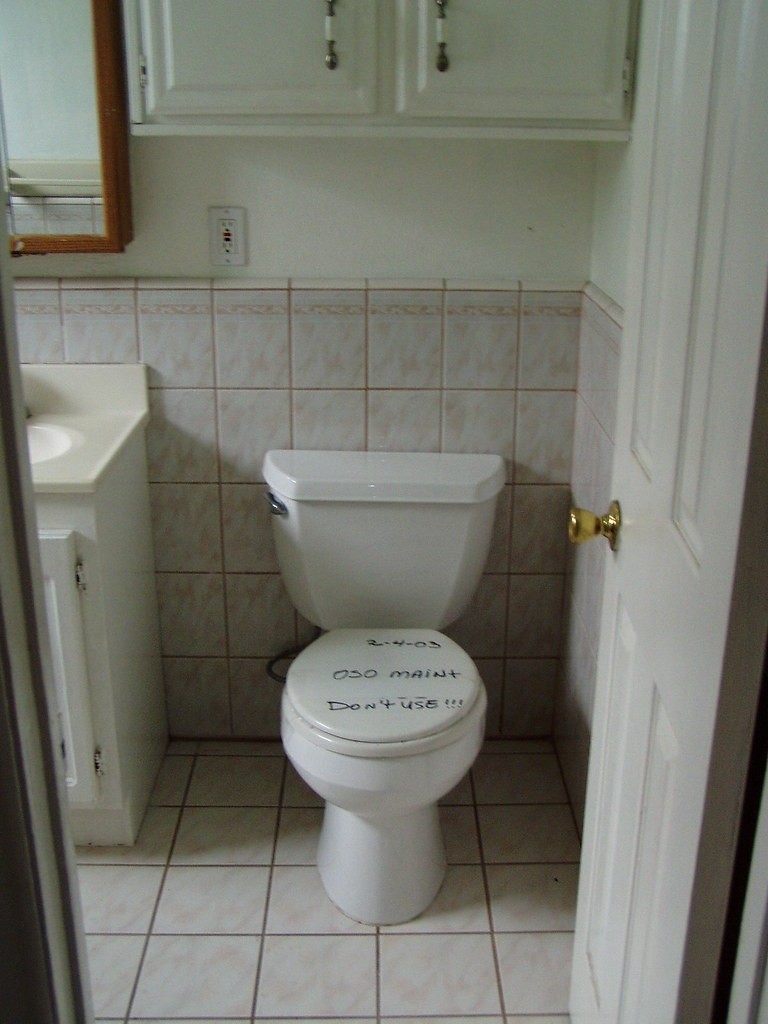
[209,206,246,266]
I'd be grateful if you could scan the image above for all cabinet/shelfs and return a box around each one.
[124,0,641,141]
[0,0,134,254]
[31,427,169,845]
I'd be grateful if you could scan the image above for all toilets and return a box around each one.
[260,449,504,924]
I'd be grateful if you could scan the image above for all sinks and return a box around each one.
[23,424,71,462]
